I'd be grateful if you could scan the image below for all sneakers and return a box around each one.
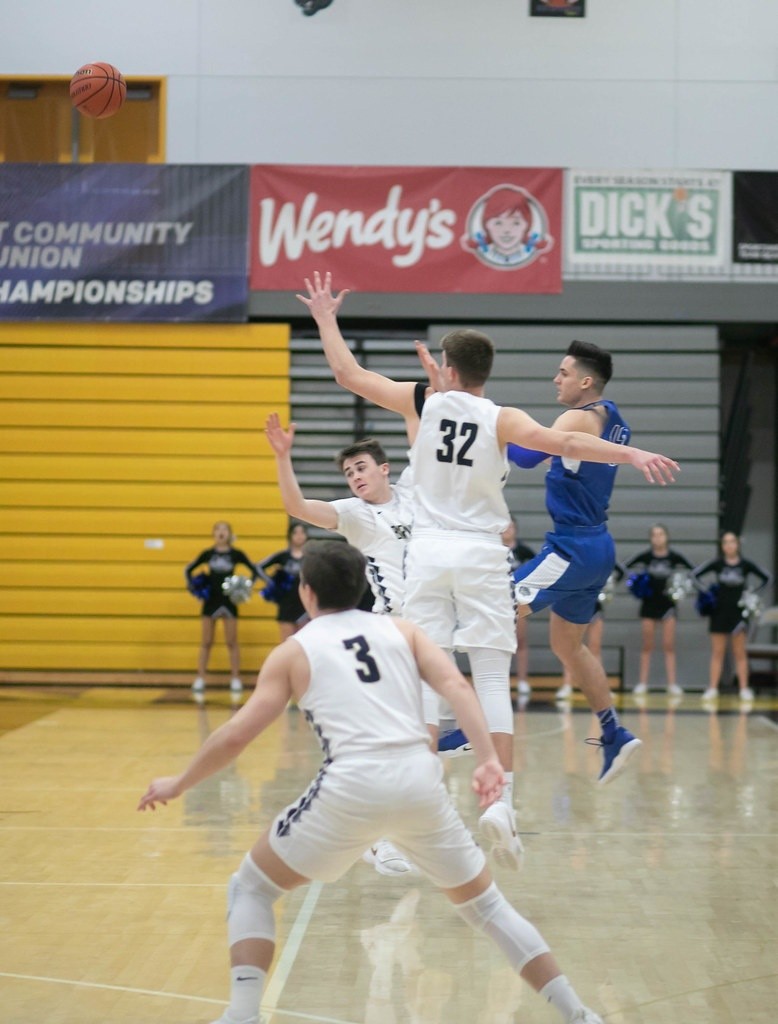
[438,729,475,757]
[478,803,527,869]
[584,725,643,784]
[208,1006,261,1024]
[570,1007,604,1024]
[364,841,411,876]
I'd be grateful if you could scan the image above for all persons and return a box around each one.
[413,333,643,789]
[183,521,274,692]
[501,514,537,695]
[254,519,311,711]
[261,413,446,760]
[137,541,610,1024]
[297,268,681,874]
[618,522,697,698]
[683,530,775,701]
[553,551,624,700]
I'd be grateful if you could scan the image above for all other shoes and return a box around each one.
[667,683,685,696]
[193,677,204,690]
[517,678,531,694]
[739,688,755,702]
[555,683,573,699]
[230,677,243,691]
[702,687,721,700]
[634,685,649,696]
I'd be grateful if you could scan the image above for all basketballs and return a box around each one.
[68,61,127,119]
